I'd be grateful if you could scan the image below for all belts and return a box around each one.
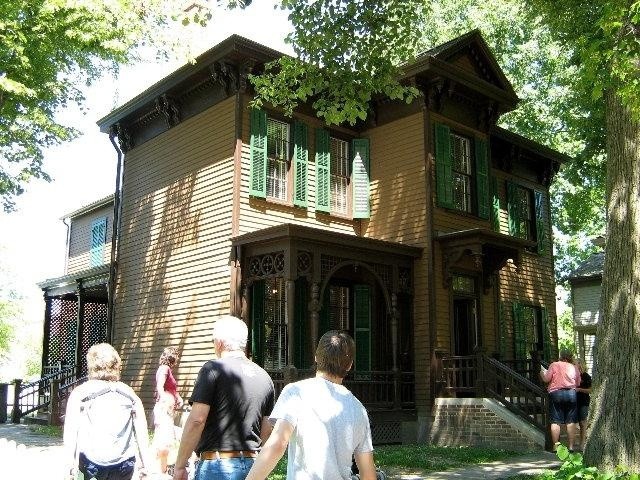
[200,450,260,461]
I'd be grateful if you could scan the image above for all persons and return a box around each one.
[540,349,582,453]
[174,315,276,480]
[62,341,153,480]
[244,330,378,480]
[149,345,184,476]
[569,357,592,452]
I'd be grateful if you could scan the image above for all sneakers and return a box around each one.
[552,447,576,455]
